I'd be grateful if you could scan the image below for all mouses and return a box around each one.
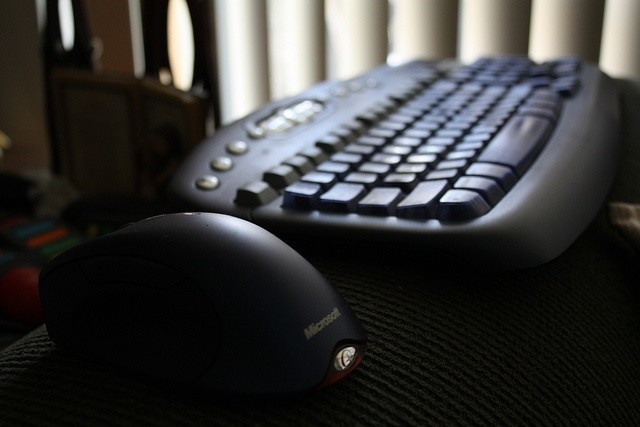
[38,209,370,397]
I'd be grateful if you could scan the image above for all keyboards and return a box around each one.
[164,53,625,274]
